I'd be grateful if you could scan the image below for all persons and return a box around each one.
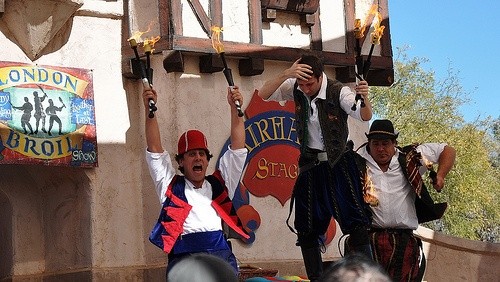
[142,83,248,282]
[257,57,457,282]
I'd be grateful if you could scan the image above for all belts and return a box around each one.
[304,151,328,166]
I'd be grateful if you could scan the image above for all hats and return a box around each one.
[177,129,207,155]
[364,120,399,141]
